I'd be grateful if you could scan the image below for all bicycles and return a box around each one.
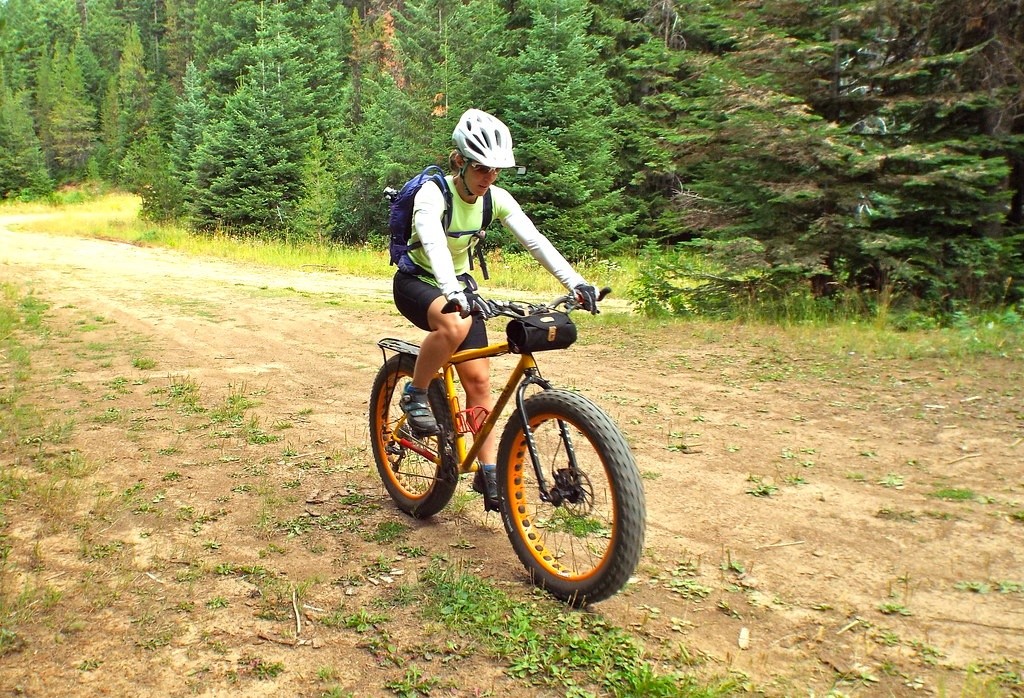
[370,288,646,608]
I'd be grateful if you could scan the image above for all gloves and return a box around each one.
[571,283,600,315]
[446,291,493,324]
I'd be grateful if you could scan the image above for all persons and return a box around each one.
[389,108,600,511]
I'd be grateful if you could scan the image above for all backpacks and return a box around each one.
[383,165,493,280]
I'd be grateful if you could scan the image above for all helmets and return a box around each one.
[451,108,516,168]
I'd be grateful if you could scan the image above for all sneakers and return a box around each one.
[472,466,504,506]
[398,382,437,433]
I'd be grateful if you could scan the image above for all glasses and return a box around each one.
[463,156,502,175]
